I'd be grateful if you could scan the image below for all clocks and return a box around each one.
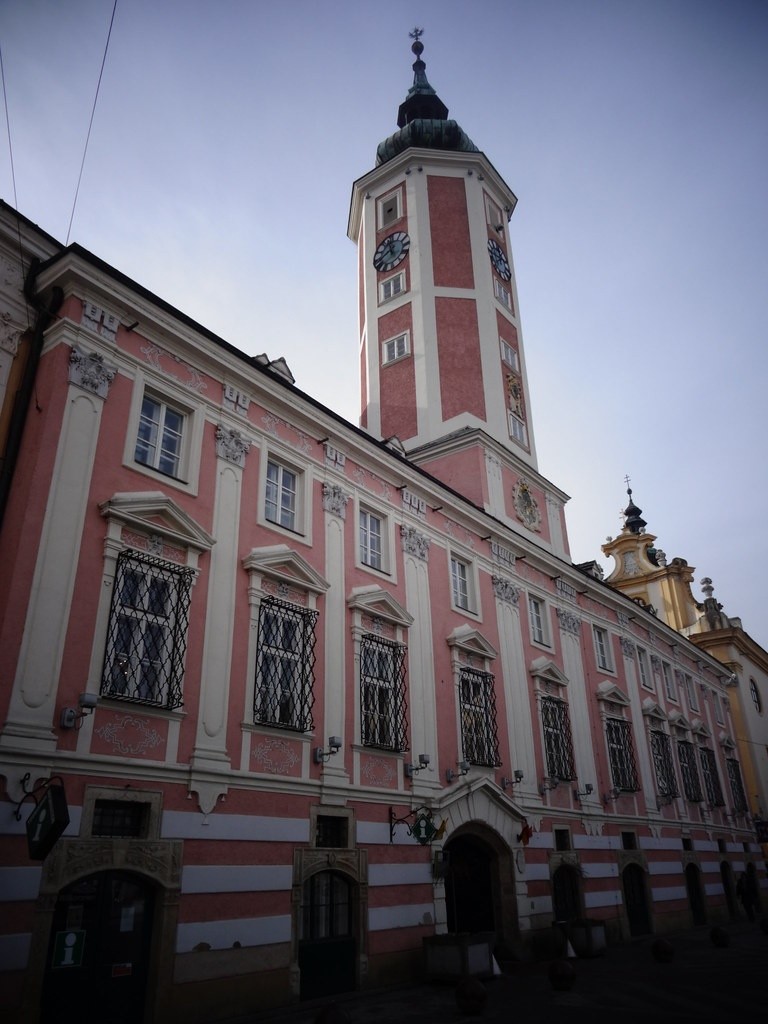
[487,239,512,281]
[373,231,410,272]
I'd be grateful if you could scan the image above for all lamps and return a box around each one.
[58,691,762,828]
